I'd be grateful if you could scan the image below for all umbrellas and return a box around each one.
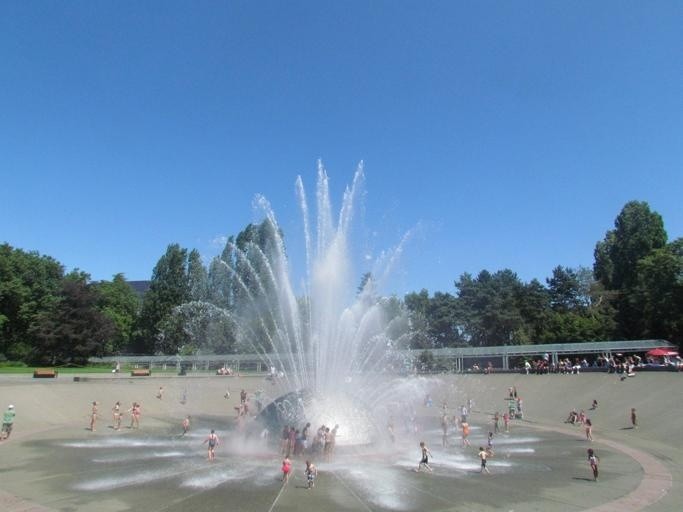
[646,348,679,356]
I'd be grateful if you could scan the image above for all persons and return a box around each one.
[281,422,339,490]
[90,362,283,462]
[0,404,16,440]
[388,385,637,482]
[472,351,682,381]
